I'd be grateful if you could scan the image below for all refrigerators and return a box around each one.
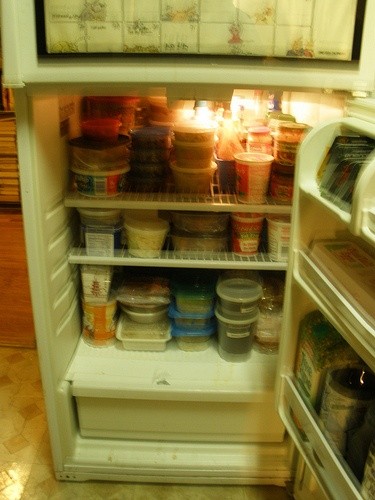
[0,0,375,500]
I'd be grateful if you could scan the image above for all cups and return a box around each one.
[233,152,274,204]
[231,213,263,256]
[267,216,291,262]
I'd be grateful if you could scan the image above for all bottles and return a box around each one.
[247,126,272,156]
[266,113,296,141]
[270,165,294,205]
[274,123,309,165]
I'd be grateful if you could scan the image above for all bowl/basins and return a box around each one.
[72,97,265,362]
